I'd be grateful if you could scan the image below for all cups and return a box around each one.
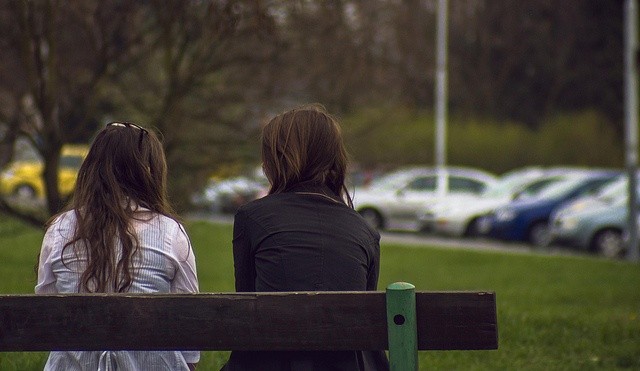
[105,121,149,154]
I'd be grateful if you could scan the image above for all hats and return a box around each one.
[1,144,91,209]
[480,168,621,248]
[420,166,563,238]
[550,173,630,258]
[344,164,500,235]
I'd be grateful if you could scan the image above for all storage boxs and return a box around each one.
[1,282,498,371]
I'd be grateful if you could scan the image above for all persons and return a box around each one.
[34,122,199,371]
[220,103,392,371]
